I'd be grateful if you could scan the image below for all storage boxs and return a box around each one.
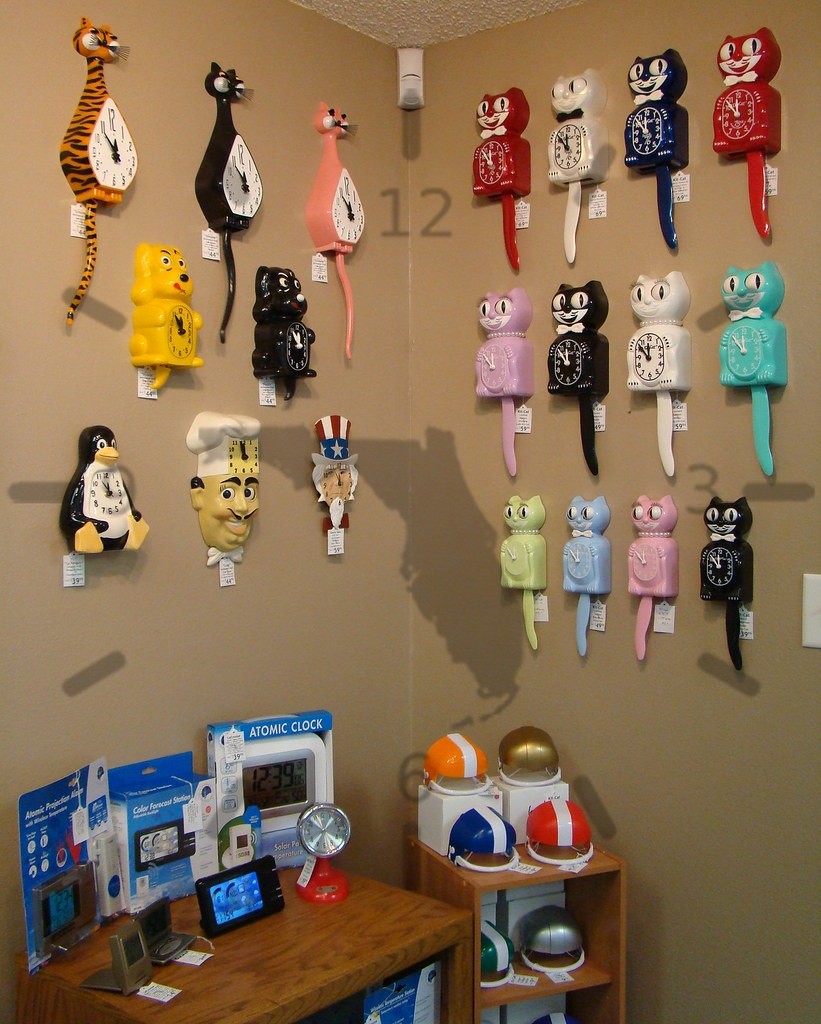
[105,751,217,917]
[417,785,503,856]
[351,959,441,1024]
[207,708,334,873]
[491,776,568,845]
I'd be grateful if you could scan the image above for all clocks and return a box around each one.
[712,28,780,240]
[296,803,352,903]
[32,861,100,957]
[715,262,787,477]
[547,281,610,475]
[626,268,692,473]
[546,67,609,263]
[562,495,611,656]
[239,733,326,834]
[624,49,689,247]
[471,289,534,475]
[57,18,364,564]
[473,87,532,270]
[499,496,545,650]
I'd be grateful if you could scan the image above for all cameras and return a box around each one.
[196,854,285,939]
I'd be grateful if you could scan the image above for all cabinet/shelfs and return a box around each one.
[406,833,630,1024]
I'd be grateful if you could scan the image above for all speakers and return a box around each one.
[395,48,424,110]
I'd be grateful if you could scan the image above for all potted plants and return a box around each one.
[628,494,678,661]
[699,497,752,671]
